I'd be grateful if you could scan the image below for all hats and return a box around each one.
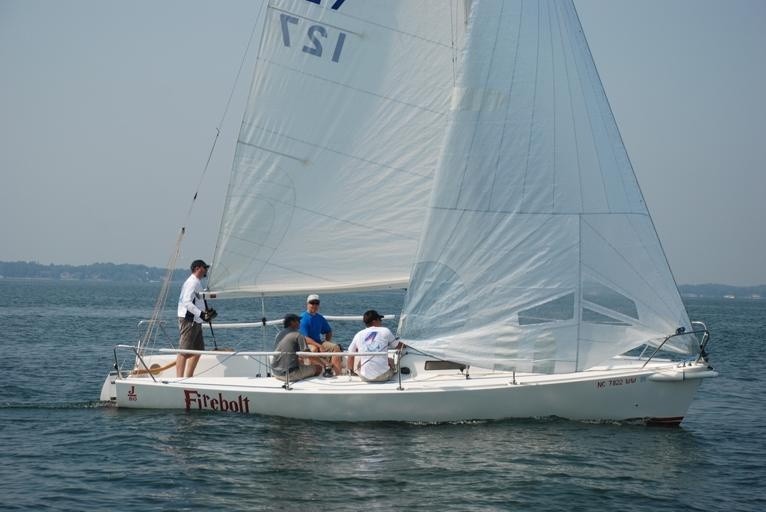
[364,310,384,323]
[307,294,319,303]
[191,259,210,269]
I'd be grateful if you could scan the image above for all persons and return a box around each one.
[347,310,401,382]
[299,295,343,375]
[176,261,218,378]
[270,314,322,381]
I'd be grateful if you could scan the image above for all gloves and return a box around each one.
[200,308,215,322]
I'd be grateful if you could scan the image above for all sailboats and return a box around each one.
[98,0,720,424]
